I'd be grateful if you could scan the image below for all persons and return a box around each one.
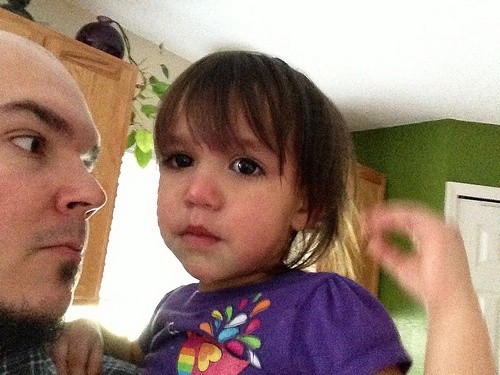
[0,26,154,375]
[42,48,499,375]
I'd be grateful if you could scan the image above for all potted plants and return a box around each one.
[74,14,175,169]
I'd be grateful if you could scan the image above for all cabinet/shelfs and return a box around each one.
[286,159,387,298]
[0,7,138,305]
